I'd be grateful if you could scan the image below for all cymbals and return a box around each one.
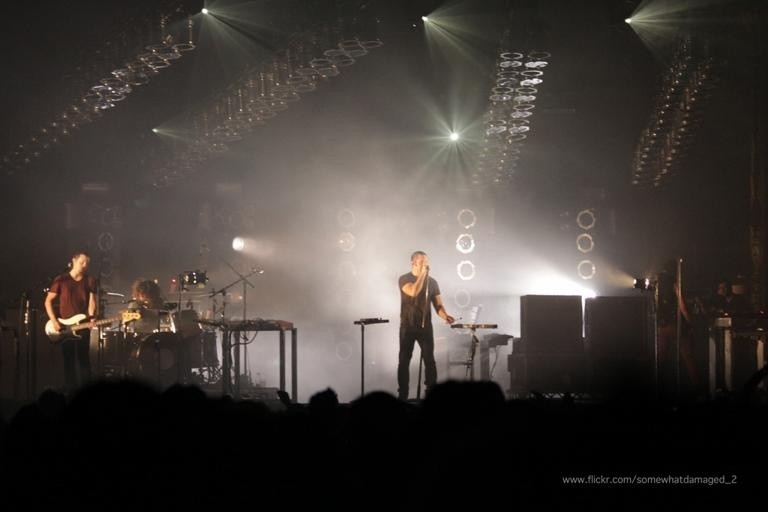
[198,292,227,298]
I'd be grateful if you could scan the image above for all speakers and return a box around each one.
[584,296,656,359]
[519,294,583,353]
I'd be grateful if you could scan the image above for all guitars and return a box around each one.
[44,310,141,343]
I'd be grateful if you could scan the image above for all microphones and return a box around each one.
[64,262,72,269]
[255,264,264,275]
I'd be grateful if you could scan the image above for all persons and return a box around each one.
[134,278,162,308]
[45,248,99,385]
[0,360,768,511]
[711,279,746,312]
[396,250,454,400]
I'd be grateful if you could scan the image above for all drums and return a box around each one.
[138,329,186,384]
[103,330,137,372]
[188,330,219,368]
[183,272,208,292]
[169,309,202,340]
